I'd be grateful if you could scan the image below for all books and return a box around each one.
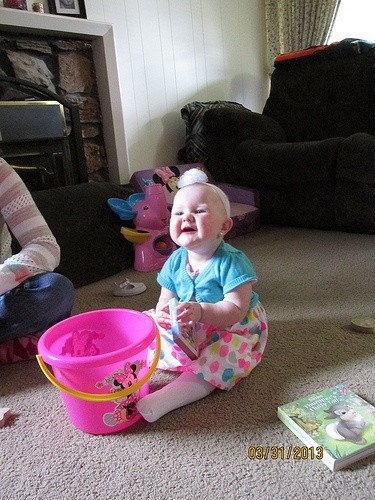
[276,385,375,473]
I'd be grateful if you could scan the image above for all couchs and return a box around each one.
[202,49,375,235]
[130,163,262,242]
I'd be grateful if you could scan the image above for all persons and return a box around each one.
[0,158,76,364]
[135,167,268,423]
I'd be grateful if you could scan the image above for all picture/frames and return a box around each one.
[48,0,87,19]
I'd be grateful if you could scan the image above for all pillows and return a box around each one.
[181,100,250,167]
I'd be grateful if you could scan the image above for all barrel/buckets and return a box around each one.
[35,308,161,437]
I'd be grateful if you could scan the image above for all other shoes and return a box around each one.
[112,278,147,296]
